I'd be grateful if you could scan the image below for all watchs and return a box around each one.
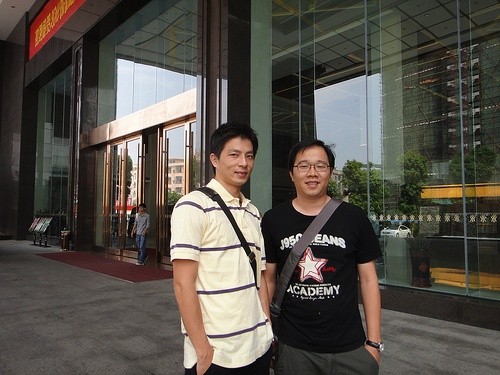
[365,340,385,352]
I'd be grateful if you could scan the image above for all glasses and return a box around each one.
[292,161,331,172]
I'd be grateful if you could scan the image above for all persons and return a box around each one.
[169,119,276,375]
[130,203,150,265]
[259,139,386,375]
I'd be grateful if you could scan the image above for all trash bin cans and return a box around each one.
[60,230,71,251]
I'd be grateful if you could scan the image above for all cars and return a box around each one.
[380,224,412,239]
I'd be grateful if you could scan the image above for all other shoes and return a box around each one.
[136,262,144,265]
[143,256,149,263]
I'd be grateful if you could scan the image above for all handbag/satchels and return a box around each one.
[269,303,283,368]
